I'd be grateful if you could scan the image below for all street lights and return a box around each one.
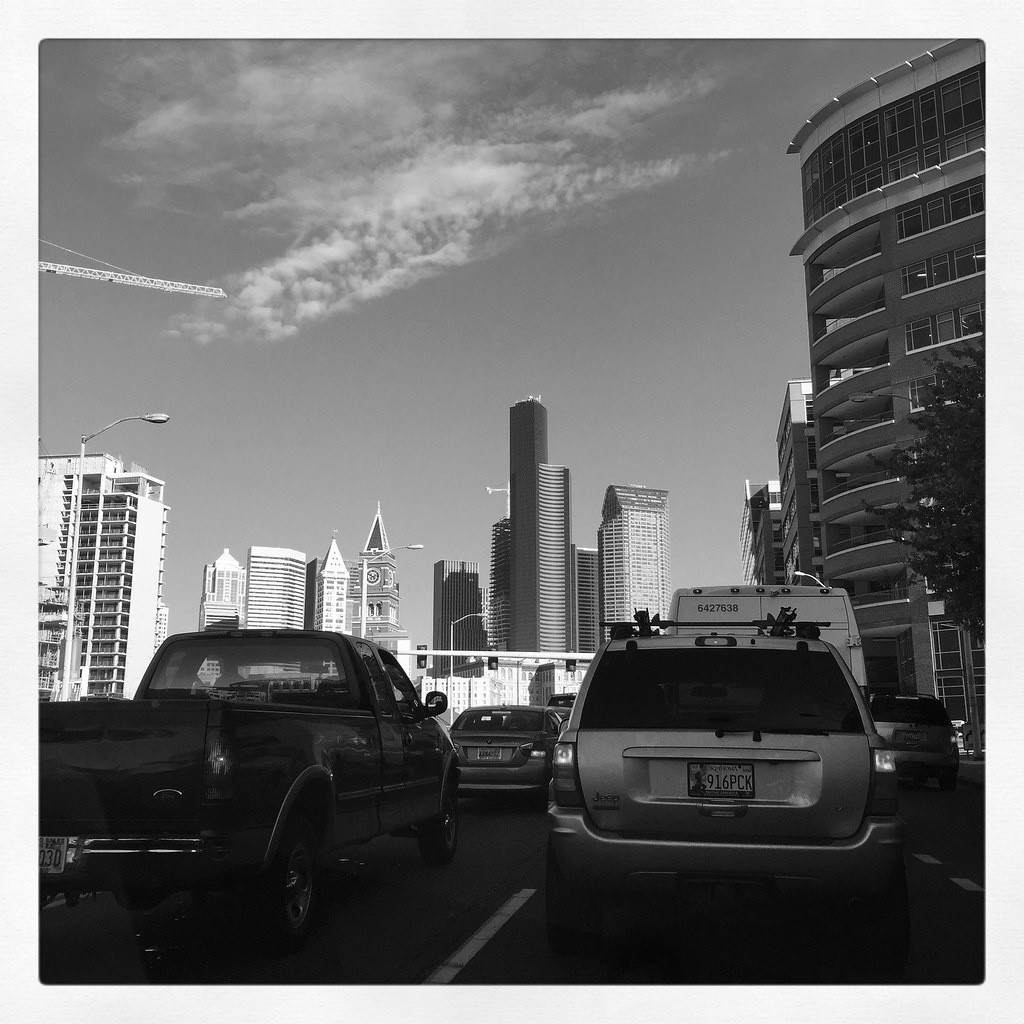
[57,412,170,696]
[359,543,425,642]
[448,612,488,731]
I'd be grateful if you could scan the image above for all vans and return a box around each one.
[666,582,872,709]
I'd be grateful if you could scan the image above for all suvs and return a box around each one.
[540,608,913,969]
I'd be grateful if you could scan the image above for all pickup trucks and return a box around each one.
[40,626,461,945]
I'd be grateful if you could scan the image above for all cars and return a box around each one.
[870,692,958,795]
[545,693,578,721]
[445,703,563,802]
